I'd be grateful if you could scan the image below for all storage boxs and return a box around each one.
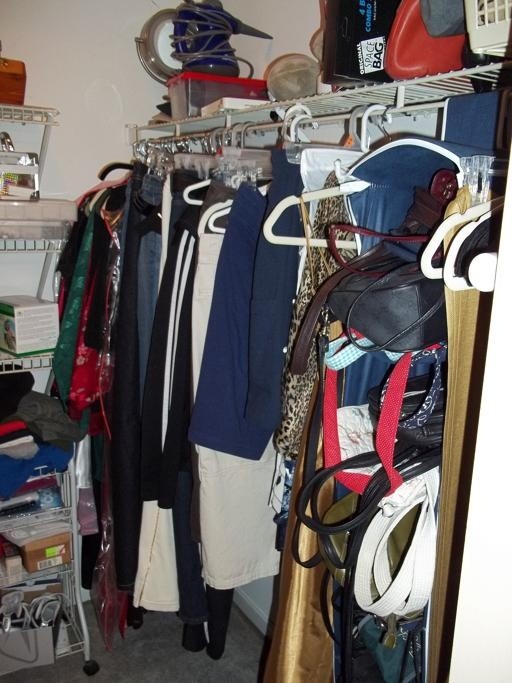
[165,72,270,121]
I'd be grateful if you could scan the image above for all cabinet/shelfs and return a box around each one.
[0,105,100,675]
